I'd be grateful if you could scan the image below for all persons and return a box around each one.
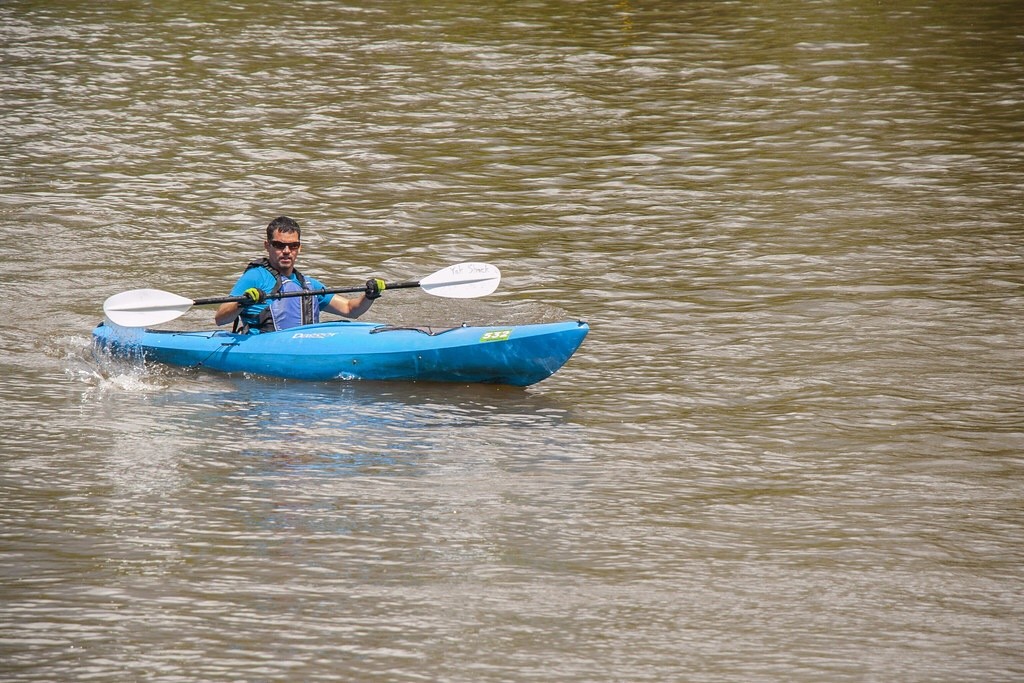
[213,215,385,339]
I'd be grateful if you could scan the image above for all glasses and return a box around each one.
[269,240,300,250]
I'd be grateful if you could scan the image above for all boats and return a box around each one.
[92,320,589,388]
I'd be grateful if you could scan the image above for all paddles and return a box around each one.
[102,261,501,326]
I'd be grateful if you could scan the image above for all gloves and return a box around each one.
[237,287,267,308]
[364,278,387,300]
